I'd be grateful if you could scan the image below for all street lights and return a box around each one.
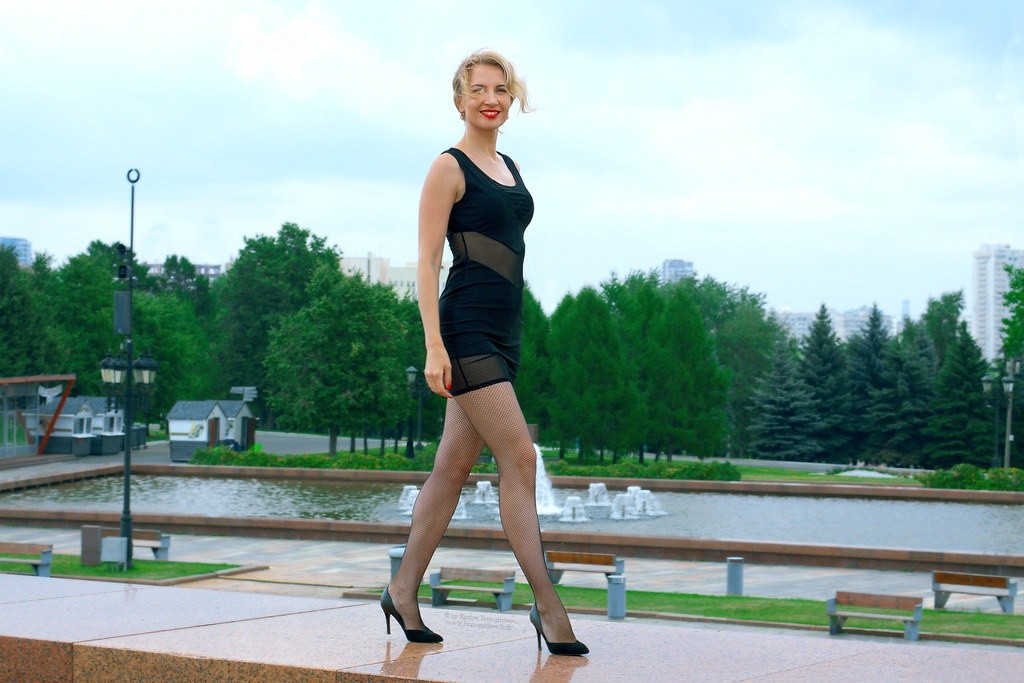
[99,339,159,569]
[1002,358,1015,469]
[404,366,419,459]
[980,374,1001,468]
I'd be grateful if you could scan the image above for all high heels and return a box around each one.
[382,586,444,643]
[530,602,590,654]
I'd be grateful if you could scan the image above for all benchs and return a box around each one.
[544,551,616,584]
[0,541,53,577]
[826,591,923,640]
[430,567,516,612]
[931,570,1018,615]
[101,527,170,561]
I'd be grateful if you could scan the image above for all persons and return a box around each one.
[378,50,590,656]
